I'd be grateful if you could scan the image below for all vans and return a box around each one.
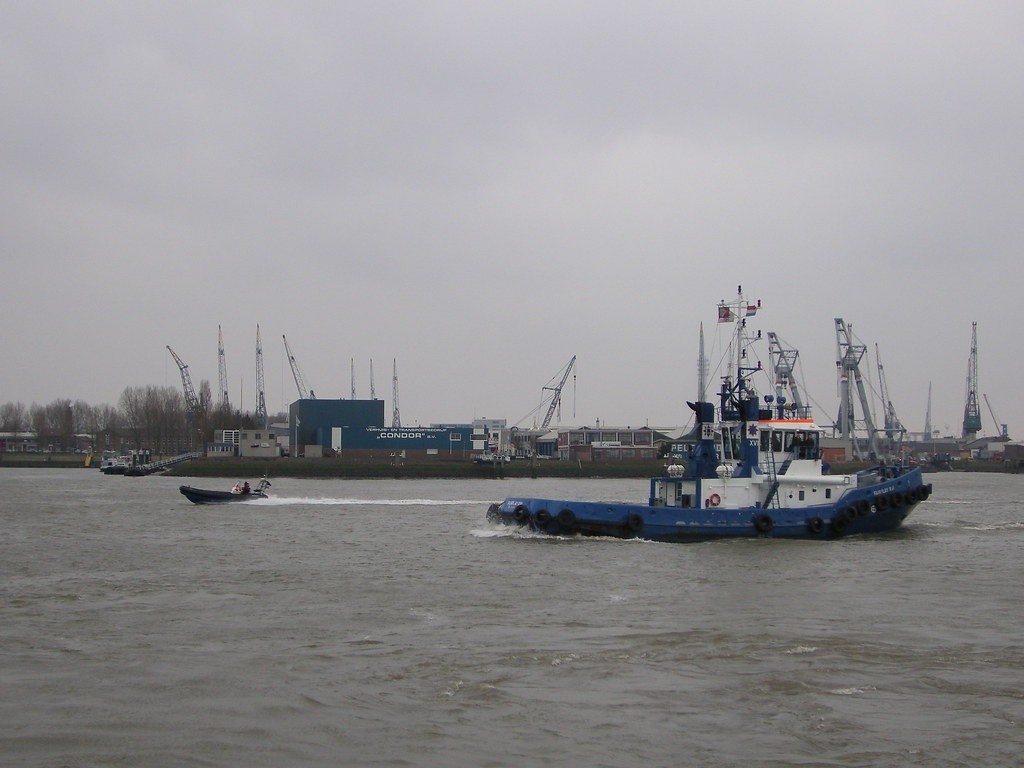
[251,442,270,448]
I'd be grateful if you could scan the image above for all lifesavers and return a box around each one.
[876,482,933,511]
[710,493,721,506]
[833,499,871,532]
[534,508,551,526]
[756,515,773,533]
[557,509,576,528]
[810,517,824,534]
[512,505,528,521]
[629,514,643,531]
[486,503,496,519]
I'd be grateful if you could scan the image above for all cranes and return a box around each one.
[165,317,1012,438]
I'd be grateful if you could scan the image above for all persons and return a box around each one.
[240,481,250,495]
[232,485,241,491]
[793,434,814,460]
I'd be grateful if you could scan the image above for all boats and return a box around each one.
[488,286,934,541]
[100,450,130,474]
[179,474,272,503]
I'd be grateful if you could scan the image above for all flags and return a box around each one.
[717,306,757,322]
